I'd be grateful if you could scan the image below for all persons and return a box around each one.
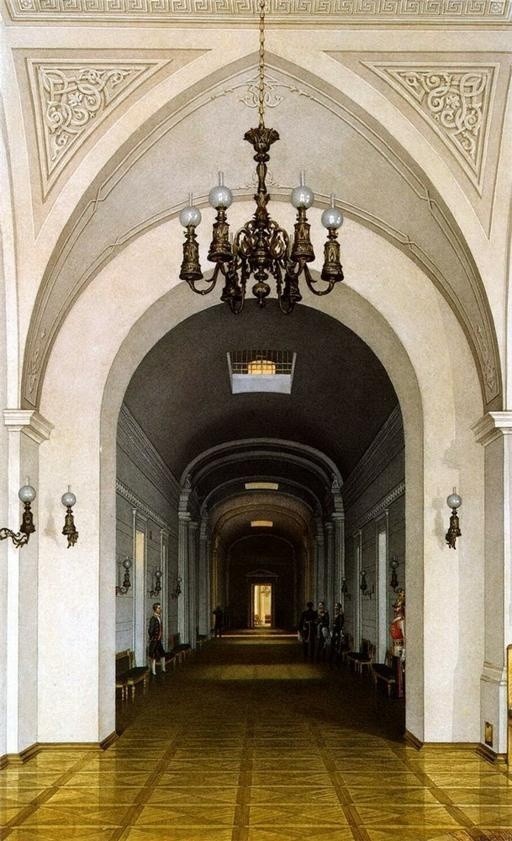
[330,602,344,648]
[147,603,169,679]
[299,602,317,657]
[315,601,329,662]
[212,606,224,638]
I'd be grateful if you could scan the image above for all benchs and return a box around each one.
[328,633,399,695]
[113,625,207,700]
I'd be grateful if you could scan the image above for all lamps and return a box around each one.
[115,556,182,598]
[0,476,80,550]
[340,554,403,599]
[446,484,462,550]
[179,0,344,316]
